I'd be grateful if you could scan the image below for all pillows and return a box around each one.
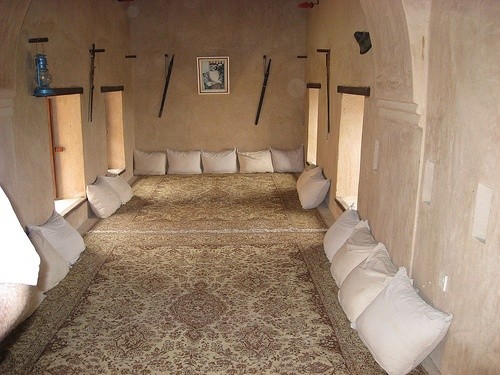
[25,230,69,293]
[269,144,304,172]
[26,209,86,268]
[296,164,331,211]
[133,148,166,175]
[166,148,202,174]
[323,208,361,263]
[102,175,135,205]
[0,283,47,340]
[86,176,122,219]
[330,219,377,289]
[201,146,238,173]
[337,242,397,323]
[355,266,453,375]
[237,147,274,173]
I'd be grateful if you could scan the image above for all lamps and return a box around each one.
[33,52,55,97]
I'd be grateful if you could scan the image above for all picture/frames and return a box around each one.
[196,55,231,96]
